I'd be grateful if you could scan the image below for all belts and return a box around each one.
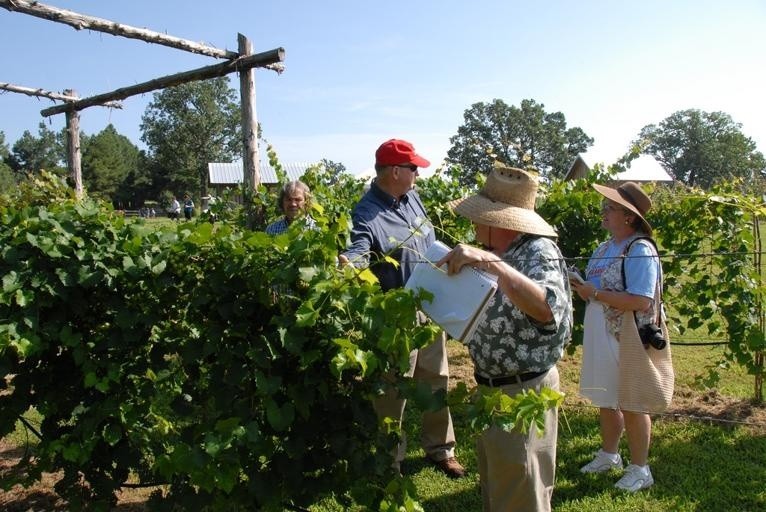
[473,369,550,387]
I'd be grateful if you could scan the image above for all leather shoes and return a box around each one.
[427,452,468,478]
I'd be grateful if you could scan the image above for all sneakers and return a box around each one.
[615,463,655,492]
[581,450,623,475]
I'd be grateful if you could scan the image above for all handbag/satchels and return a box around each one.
[619,307,674,413]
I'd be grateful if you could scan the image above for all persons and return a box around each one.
[182,194,195,222]
[432,163,576,511]
[336,136,466,480]
[568,180,666,494]
[137,206,156,218]
[168,195,181,221]
[264,179,322,236]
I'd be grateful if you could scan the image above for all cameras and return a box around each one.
[639,324,667,350]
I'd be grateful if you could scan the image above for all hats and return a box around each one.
[446,167,558,237]
[592,181,653,236]
[376,139,431,167]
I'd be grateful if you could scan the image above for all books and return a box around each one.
[566,269,586,287]
[402,239,500,348]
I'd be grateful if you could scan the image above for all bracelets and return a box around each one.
[592,286,600,302]
